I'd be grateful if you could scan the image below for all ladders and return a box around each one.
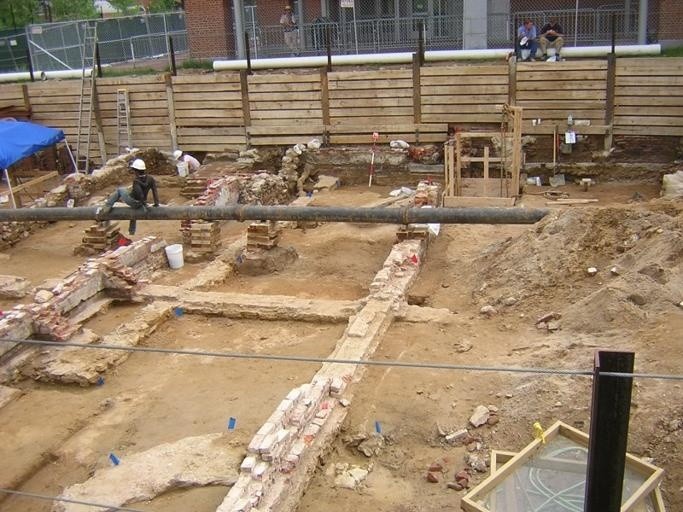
[76,22,97,175]
[117,88,131,156]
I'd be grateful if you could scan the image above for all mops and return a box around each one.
[368,131,379,188]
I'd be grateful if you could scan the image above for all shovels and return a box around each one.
[549,114,576,188]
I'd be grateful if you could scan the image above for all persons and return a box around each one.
[280,5,301,58]
[97,159,159,235]
[536,14,564,63]
[173,149,200,176]
[515,19,536,62]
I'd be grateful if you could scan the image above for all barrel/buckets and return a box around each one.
[177,160,189,175]
[526,176,541,186]
[661,173,683,196]
[165,243,184,268]
[564,132,575,143]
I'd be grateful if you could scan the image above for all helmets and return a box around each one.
[172,149,183,160]
[130,158,147,171]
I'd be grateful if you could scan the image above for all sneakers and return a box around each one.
[516,54,562,62]
